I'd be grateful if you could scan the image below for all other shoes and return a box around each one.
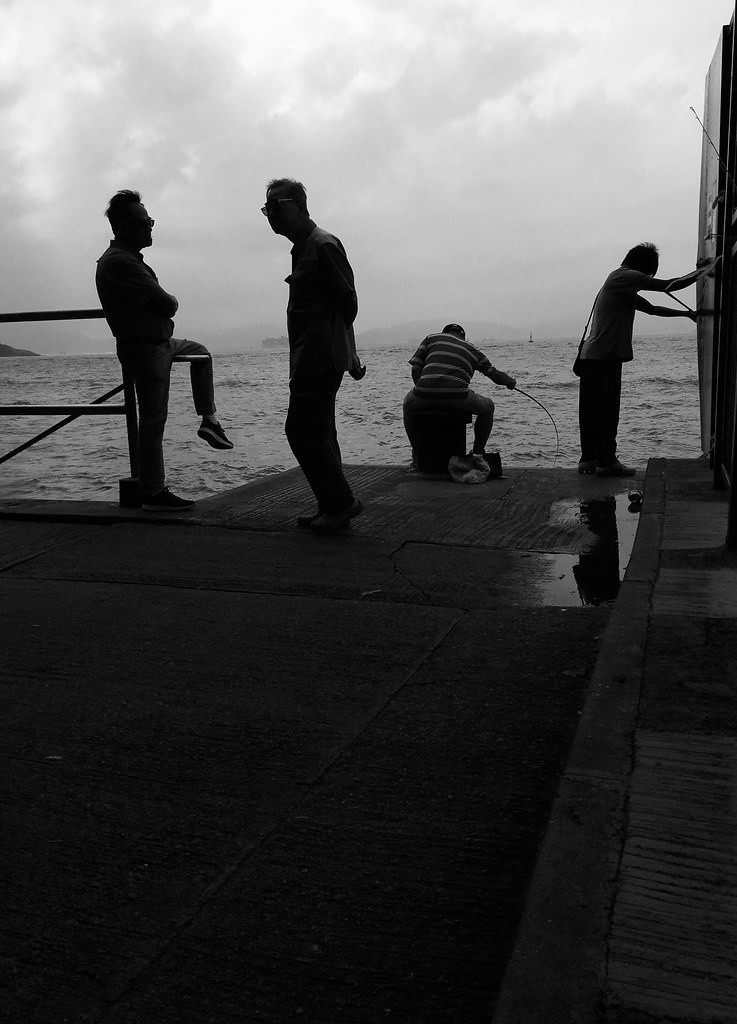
[410,462,417,468]
[578,461,596,474]
[311,499,363,531]
[596,455,636,477]
[142,486,197,511]
[297,511,327,527]
[197,418,234,449]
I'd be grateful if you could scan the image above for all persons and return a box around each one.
[261,179,366,534]
[403,324,516,467]
[96,189,234,512]
[578,242,708,476]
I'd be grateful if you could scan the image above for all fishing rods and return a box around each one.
[512,384,564,467]
[662,289,697,322]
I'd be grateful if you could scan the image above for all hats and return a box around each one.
[442,323,465,341]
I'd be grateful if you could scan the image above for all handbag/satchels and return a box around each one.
[573,340,586,376]
[573,565,585,599]
[485,452,503,478]
[448,450,493,485]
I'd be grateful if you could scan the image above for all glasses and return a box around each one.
[261,199,294,216]
[140,220,154,229]
